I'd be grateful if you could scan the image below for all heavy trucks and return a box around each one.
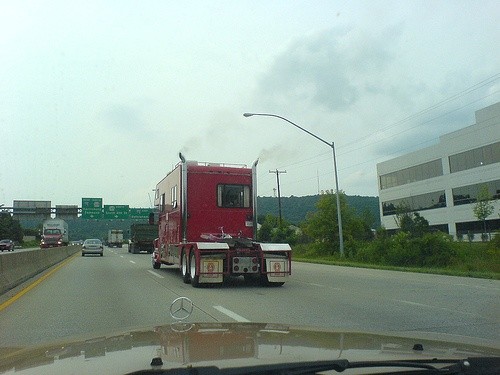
[148,151,292,288]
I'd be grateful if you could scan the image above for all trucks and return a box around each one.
[40,218,69,248]
[127,223,159,254]
[108,230,124,249]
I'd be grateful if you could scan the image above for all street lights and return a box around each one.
[243,113,345,258]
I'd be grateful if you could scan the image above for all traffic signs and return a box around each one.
[81,198,102,219]
[104,205,129,220]
[129,208,153,221]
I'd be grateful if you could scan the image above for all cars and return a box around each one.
[81,239,104,256]
[0,239,15,252]
